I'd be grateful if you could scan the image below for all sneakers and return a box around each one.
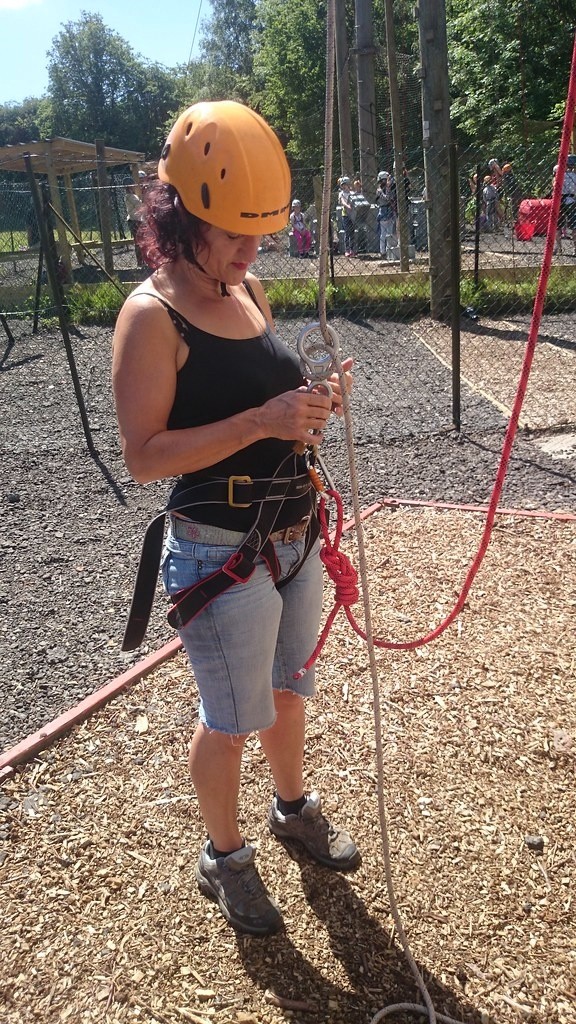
[268,790,362,870]
[195,840,284,936]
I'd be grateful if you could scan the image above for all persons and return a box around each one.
[460,153,576,256]
[123,178,147,267]
[110,100,362,937]
[290,160,414,260]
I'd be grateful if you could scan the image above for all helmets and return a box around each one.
[489,159,497,171]
[292,199,301,207]
[567,154,576,165]
[393,161,405,169]
[340,177,350,187]
[138,170,147,178]
[377,171,389,183]
[157,101,292,236]
[122,177,135,185]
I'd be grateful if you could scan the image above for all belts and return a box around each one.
[171,508,312,546]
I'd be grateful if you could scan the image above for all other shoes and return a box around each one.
[345,252,350,257]
[350,250,357,258]
[305,252,310,258]
[299,253,304,259]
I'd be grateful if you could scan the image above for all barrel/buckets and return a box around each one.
[517,223,534,240]
